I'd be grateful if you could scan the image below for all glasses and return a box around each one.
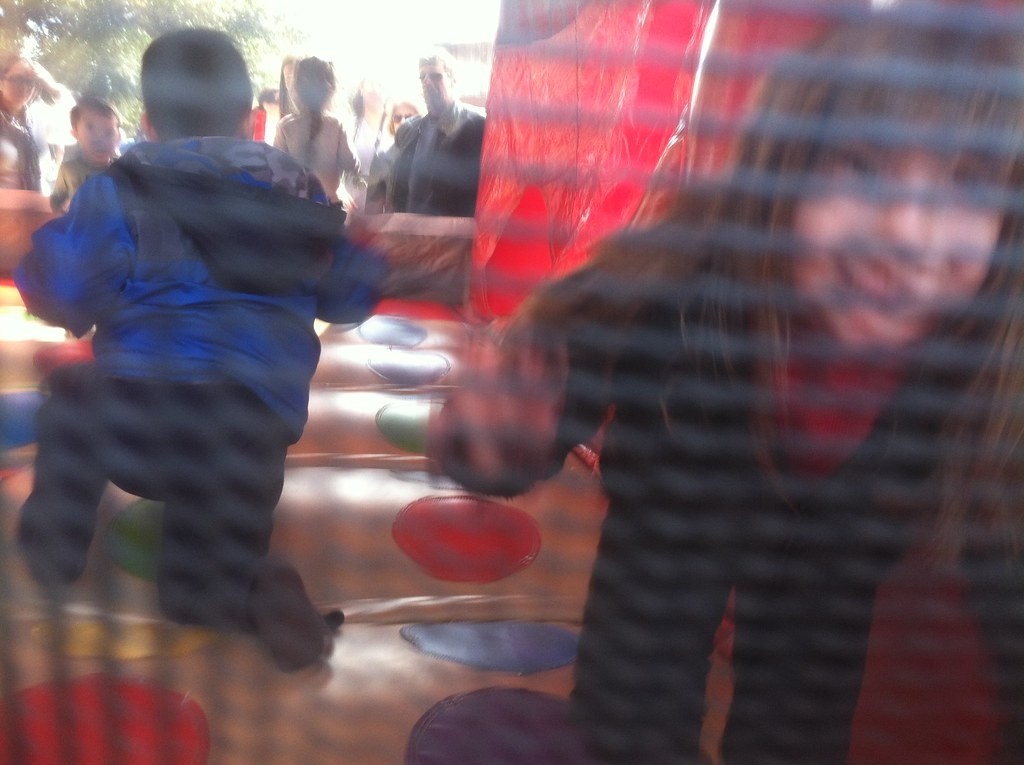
[420,71,448,80]
[393,115,412,123]
[4,75,36,90]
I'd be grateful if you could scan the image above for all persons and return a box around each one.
[13,29,390,672]
[273,57,368,208]
[48,100,120,338]
[430,22,1024,764]
[1,53,488,218]
